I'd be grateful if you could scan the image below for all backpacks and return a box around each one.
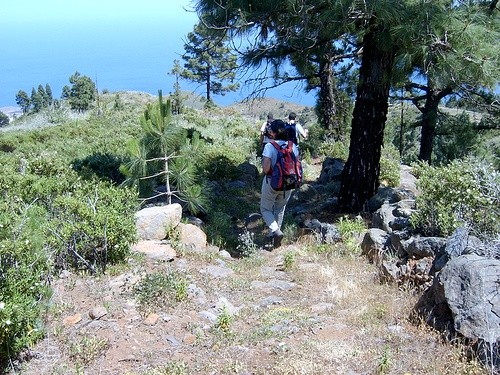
[264,121,272,136]
[270,140,303,191]
[285,122,296,142]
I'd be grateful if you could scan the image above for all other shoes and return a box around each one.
[264,229,283,248]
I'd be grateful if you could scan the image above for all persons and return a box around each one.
[261,112,276,153]
[259,118,300,249]
[286,112,310,163]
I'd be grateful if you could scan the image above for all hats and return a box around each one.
[271,120,284,131]
